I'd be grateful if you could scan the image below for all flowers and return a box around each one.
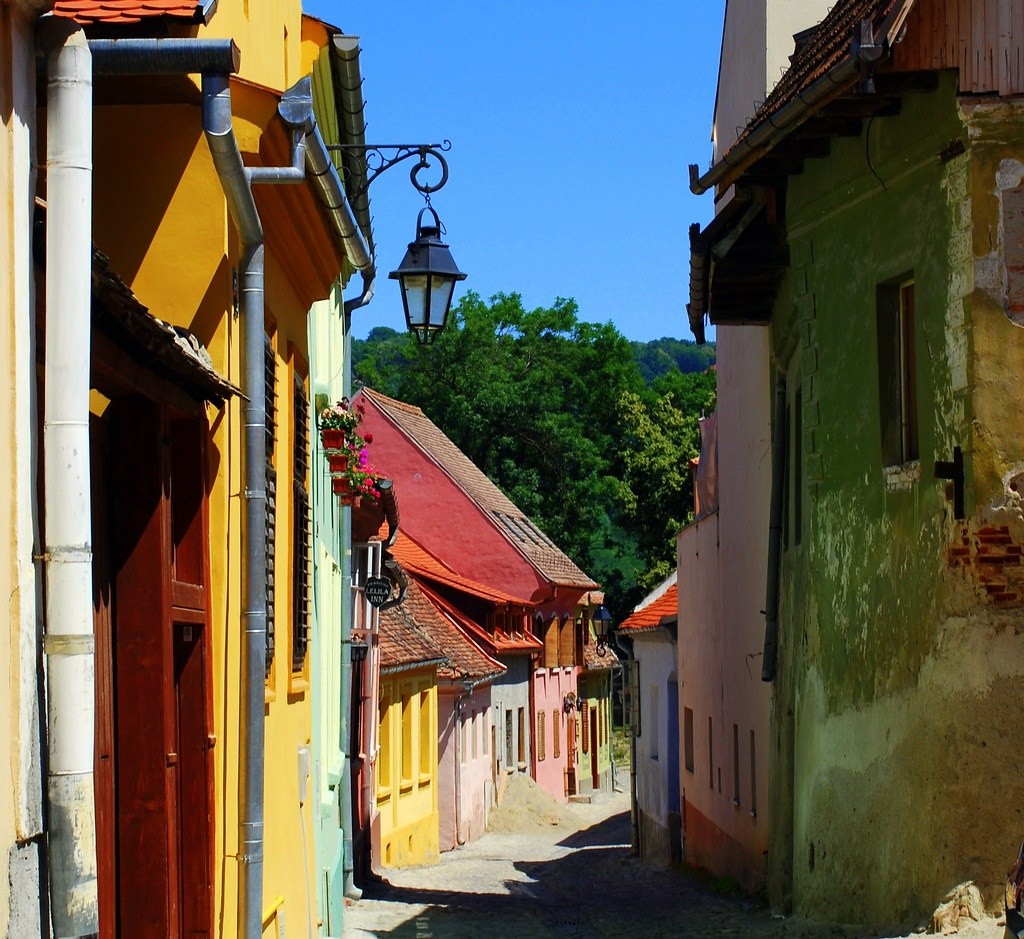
[317,393,382,506]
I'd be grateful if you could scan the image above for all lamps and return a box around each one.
[592,605,612,656]
[321,141,466,352]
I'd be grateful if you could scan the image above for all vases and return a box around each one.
[330,454,348,472]
[320,430,344,450]
[342,495,360,506]
[333,477,350,493]
